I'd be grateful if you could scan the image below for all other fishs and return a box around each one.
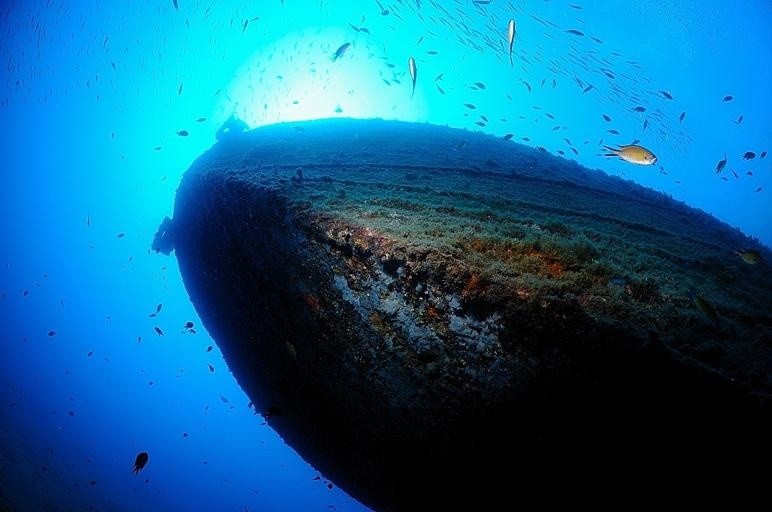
[0,211,197,508]
[1,0,772,193]
[201,342,231,465]
